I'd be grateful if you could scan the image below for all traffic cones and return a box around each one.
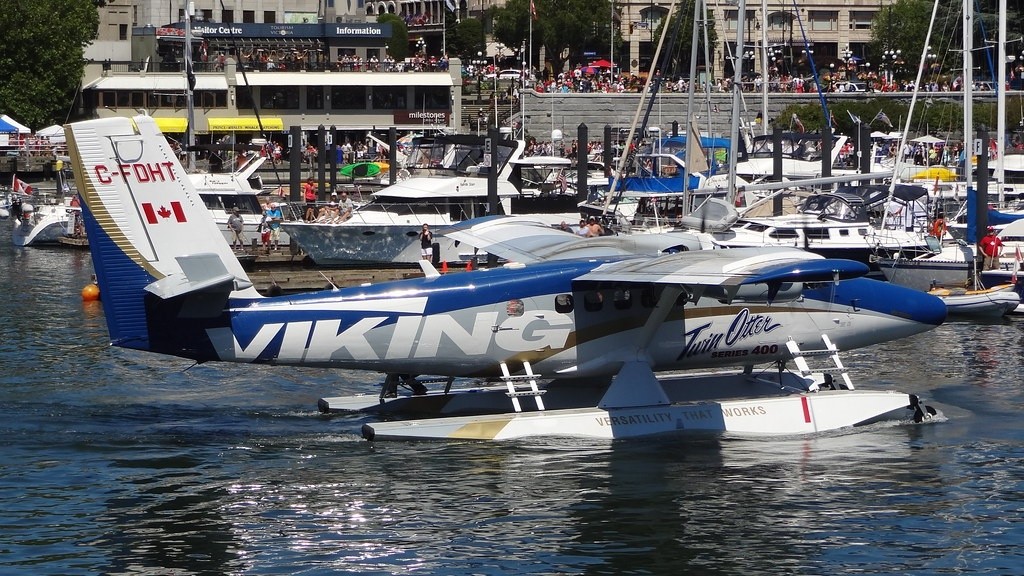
[441,261,448,272]
[465,261,472,272]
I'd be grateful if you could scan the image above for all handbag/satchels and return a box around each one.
[256,223,262,233]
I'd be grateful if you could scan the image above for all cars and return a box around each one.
[848,82,866,91]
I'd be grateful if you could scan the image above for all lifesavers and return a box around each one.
[934,219,947,236]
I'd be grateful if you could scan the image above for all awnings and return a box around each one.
[131,118,188,133]
[207,117,283,131]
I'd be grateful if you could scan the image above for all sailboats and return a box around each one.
[576,0,1024,290]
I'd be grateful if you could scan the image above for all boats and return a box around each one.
[280,133,611,266]
[940,292,1021,317]
[925,278,1015,296]
[0,188,88,246]
[181,156,291,245]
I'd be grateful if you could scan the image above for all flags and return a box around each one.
[875,112,894,127]
[444,0,457,12]
[531,1,538,21]
[829,110,840,128]
[848,111,862,124]
[792,113,806,135]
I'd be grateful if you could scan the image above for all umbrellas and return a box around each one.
[580,66,598,73]
[590,59,611,67]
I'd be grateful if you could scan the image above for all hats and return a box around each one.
[331,192,337,197]
[233,206,240,212]
[986,226,994,232]
[580,218,585,222]
[589,216,595,221]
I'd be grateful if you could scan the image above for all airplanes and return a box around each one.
[64,115,951,441]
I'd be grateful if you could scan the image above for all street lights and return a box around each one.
[471,52,488,105]
[829,63,834,92]
[881,49,902,92]
[416,37,426,53]
[921,46,937,85]
[840,44,853,81]
[745,43,814,92]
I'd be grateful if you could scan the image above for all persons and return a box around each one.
[978,226,1003,271]
[258,211,272,253]
[1,0,1023,237]
[267,204,282,251]
[227,207,244,250]
[418,223,432,263]
